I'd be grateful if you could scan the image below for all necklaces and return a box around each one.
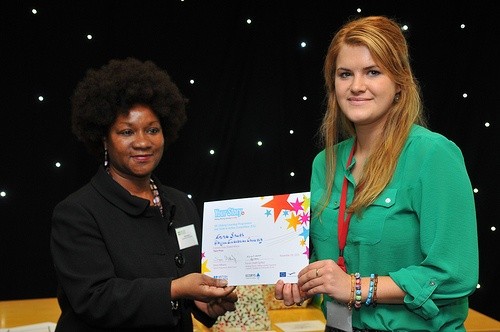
[147,179,164,218]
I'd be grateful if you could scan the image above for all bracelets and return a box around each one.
[171,299,178,311]
[348,273,378,309]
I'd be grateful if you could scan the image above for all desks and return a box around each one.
[0,285,500,332]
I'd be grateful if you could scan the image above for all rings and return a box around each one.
[316,269,319,277]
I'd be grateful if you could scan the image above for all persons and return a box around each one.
[50,58,237,332]
[274,17,477,332]
